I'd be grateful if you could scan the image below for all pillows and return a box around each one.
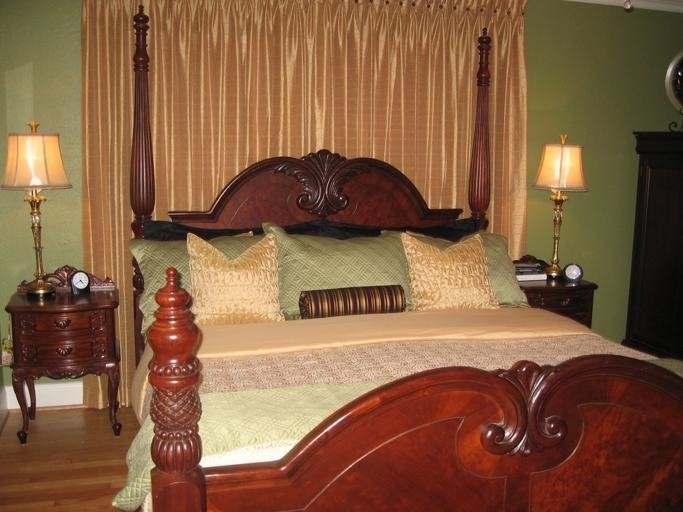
[183,230,287,330]
[394,231,502,313]
[376,222,533,311]
[255,220,416,319]
[117,233,266,341]
[294,285,407,321]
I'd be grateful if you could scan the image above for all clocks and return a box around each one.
[67,269,91,295]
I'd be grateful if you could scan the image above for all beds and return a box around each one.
[91,2,680,512]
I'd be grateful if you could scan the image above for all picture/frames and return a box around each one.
[664,51,683,117]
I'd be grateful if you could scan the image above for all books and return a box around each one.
[515,262,547,281]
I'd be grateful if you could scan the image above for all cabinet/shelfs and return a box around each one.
[621,120,682,366]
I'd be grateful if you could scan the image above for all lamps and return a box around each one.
[1,119,73,301]
[530,130,590,279]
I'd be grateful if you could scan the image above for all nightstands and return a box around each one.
[2,263,126,445]
[508,254,597,331]
